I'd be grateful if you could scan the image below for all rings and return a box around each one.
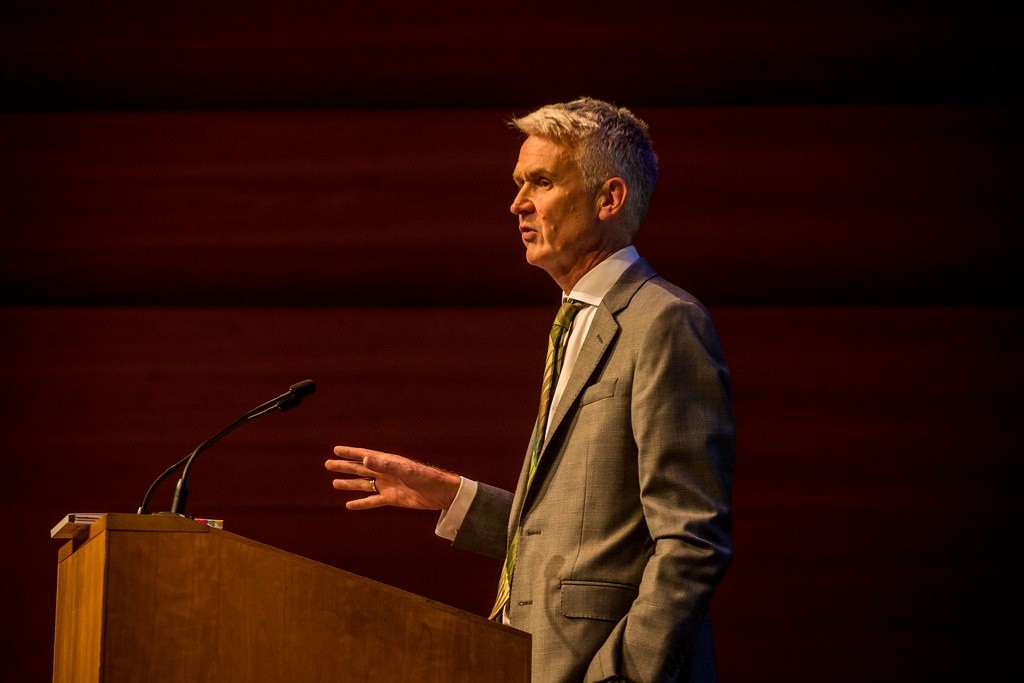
[370,479,378,493]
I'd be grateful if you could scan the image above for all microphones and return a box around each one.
[137,379,317,517]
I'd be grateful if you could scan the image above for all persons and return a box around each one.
[324,94,737,683]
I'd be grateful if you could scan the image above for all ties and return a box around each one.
[486,298,588,620]
